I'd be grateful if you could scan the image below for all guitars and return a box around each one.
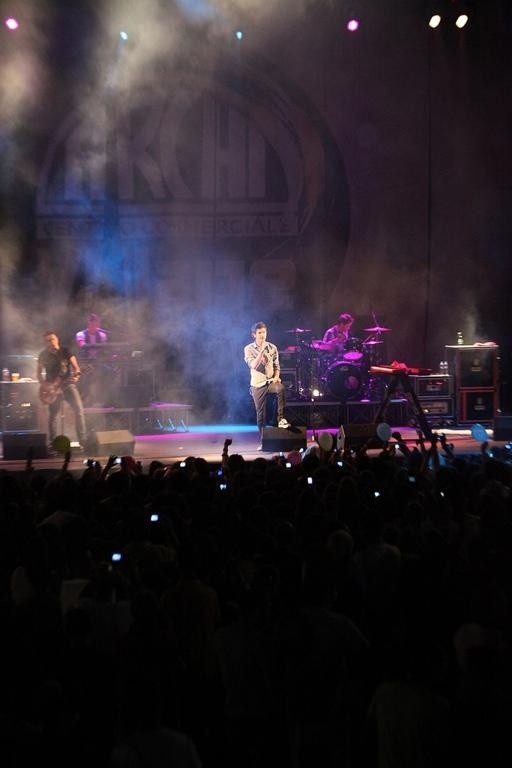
[37,364,94,406]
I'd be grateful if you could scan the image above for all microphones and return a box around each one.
[266,347,269,353]
[95,325,107,335]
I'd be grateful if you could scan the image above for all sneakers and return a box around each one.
[278,418,292,428]
[257,444,262,451]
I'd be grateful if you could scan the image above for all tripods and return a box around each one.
[374,376,438,443]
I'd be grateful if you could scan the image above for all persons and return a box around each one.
[323,313,354,344]
[76,313,108,348]
[1,432,512,573]
[37,331,87,450]
[244,322,292,451]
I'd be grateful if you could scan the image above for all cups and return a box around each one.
[11,373,20,382]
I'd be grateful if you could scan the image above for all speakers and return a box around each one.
[261,425,306,450]
[492,415,511,441]
[2,430,48,460]
[88,430,135,457]
[337,421,390,449]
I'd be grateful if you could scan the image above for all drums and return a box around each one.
[309,339,323,349]
[320,342,339,357]
[344,351,365,368]
[345,337,363,353]
[324,361,364,400]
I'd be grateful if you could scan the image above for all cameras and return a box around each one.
[111,550,123,562]
[306,476,313,484]
[149,513,161,521]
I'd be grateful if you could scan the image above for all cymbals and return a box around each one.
[363,327,390,333]
[284,328,311,333]
[362,340,384,344]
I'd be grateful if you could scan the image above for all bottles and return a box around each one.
[439,361,444,376]
[444,361,449,376]
